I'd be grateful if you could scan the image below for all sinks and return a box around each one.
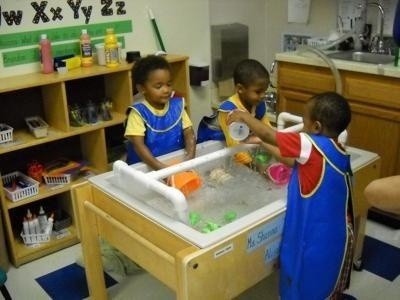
[327,50,395,65]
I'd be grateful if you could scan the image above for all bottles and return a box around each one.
[21,205,59,242]
[104,28,121,68]
[79,29,93,68]
[39,34,54,73]
[70,100,114,127]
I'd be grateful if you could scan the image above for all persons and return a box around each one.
[124,55,196,177]
[196,59,277,146]
[226,92,358,300]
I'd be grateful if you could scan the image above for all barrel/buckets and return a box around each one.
[165,160,202,197]
[266,163,291,185]
[226,109,249,141]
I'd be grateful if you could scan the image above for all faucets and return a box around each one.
[357,2,385,53]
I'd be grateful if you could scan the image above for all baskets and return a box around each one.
[0,171,41,203]
[0,123,15,144]
[42,172,72,186]
[24,115,50,140]
[19,224,54,246]
[53,208,72,232]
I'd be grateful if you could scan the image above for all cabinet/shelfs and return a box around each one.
[1,56,192,269]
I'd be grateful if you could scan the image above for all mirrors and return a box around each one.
[211,22,249,101]
